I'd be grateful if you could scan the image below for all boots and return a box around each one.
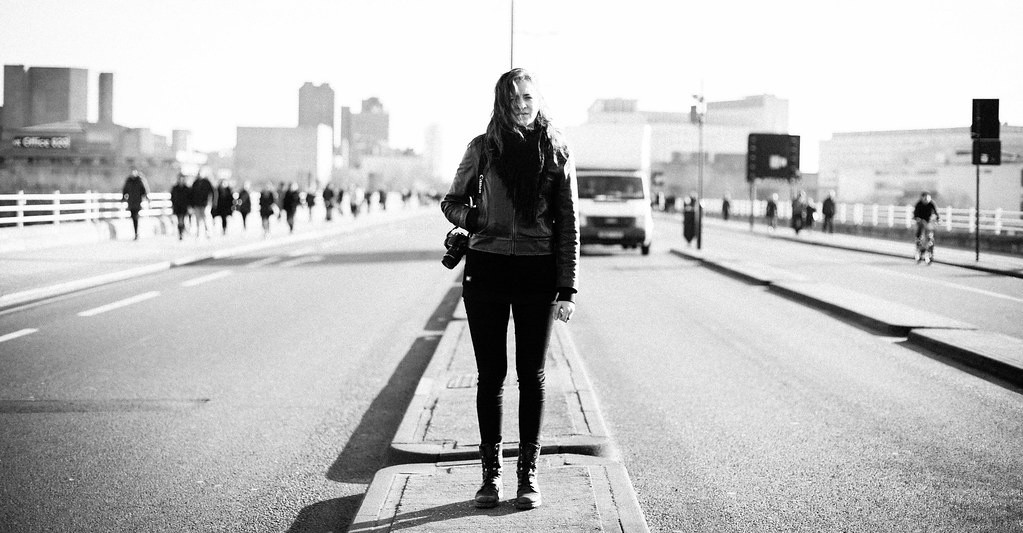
[475,436,503,508]
[516,443,541,508]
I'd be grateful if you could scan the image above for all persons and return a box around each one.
[171,169,443,240]
[656,191,730,221]
[123,169,152,240]
[441,68,580,509]
[766,191,835,234]
[914,192,940,245]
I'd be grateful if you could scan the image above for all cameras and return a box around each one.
[441,232,470,270]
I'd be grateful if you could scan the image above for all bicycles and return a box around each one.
[914,219,941,266]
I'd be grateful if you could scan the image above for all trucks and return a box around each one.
[562,122,664,255]
